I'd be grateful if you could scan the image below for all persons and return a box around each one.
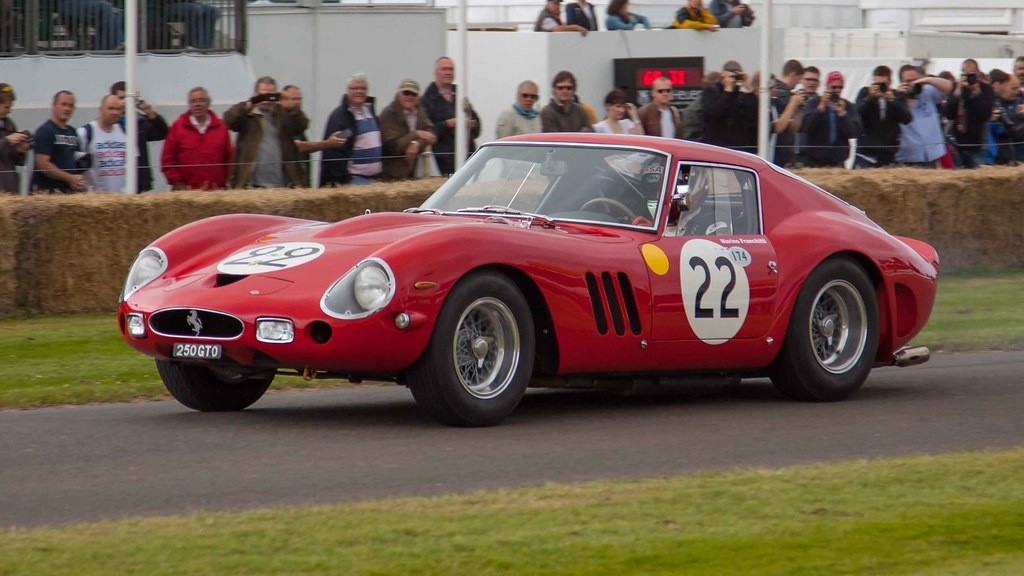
[379,78,437,181]
[566,0,598,31]
[420,57,481,176]
[591,88,645,135]
[318,73,385,188]
[497,80,544,163]
[273,85,348,188]
[223,76,308,189]
[681,55,1024,169]
[57,0,124,51]
[112,81,169,194]
[535,0,588,37]
[540,70,596,181]
[0,83,30,194]
[709,0,755,28]
[605,0,651,31]
[637,77,684,139]
[76,95,141,192]
[161,87,234,192]
[672,0,720,31]
[32,90,87,194]
[161,0,217,55]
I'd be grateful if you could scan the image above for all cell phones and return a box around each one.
[264,93,280,102]
[336,128,353,139]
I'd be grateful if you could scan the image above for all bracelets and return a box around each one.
[410,141,418,144]
[635,120,642,124]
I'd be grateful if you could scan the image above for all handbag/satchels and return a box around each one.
[415,151,443,177]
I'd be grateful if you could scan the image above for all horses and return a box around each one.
[187,310,203,336]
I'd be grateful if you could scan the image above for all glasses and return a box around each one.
[803,78,818,81]
[554,85,573,90]
[349,87,366,91]
[830,85,843,89]
[656,89,671,93]
[402,91,417,97]
[521,93,537,100]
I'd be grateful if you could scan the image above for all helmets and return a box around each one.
[641,154,710,226]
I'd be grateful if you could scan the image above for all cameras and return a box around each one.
[873,82,887,93]
[829,90,839,102]
[23,129,38,149]
[907,83,922,99]
[730,70,743,80]
[961,73,977,85]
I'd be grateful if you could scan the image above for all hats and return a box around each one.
[399,78,421,94]
[0,82,15,101]
[723,60,741,70]
[547,0,563,3]
[827,71,844,84]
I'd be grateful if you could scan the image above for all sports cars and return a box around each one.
[116,131,943,429]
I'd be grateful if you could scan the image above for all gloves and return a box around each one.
[633,216,653,227]
